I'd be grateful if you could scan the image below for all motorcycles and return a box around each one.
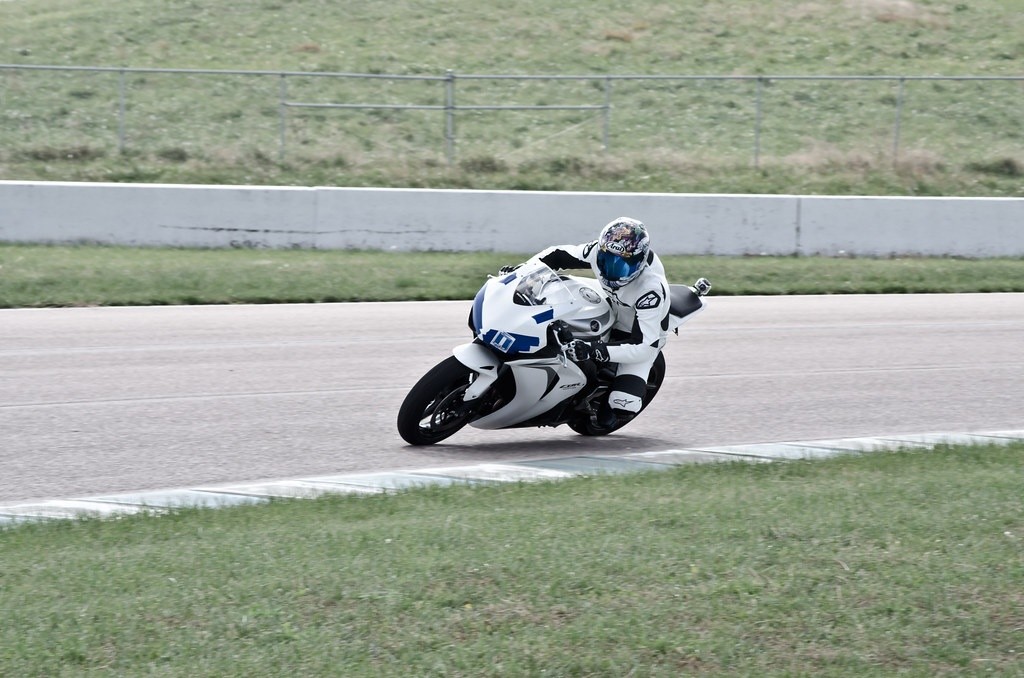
[396,256,713,446]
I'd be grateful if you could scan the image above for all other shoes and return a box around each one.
[597,396,619,428]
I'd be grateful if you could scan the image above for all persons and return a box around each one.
[499,217,671,430]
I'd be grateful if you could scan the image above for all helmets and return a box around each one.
[595,216,651,287]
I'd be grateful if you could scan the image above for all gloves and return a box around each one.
[568,339,593,362]
[499,263,524,276]
